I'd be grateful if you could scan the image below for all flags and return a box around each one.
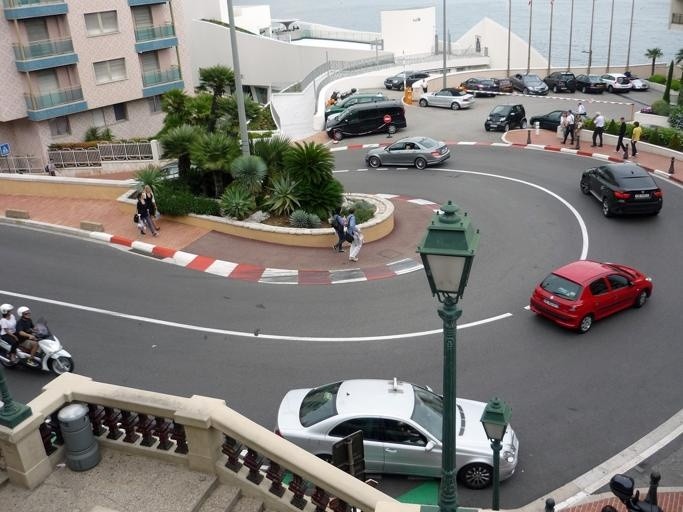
[528,1,531,6]
[550,0,554,5]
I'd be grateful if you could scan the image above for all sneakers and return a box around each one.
[11,352,16,361]
[26,357,39,367]
[141,226,160,237]
[333,245,344,252]
[349,256,358,261]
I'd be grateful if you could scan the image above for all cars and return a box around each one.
[418,87,475,110]
[526,107,573,133]
[460,77,497,98]
[489,76,512,95]
[575,73,605,95]
[578,161,665,220]
[363,131,453,171]
[529,257,655,337]
[269,374,520,489]
[509,71,549,96]
[483,102,530,135]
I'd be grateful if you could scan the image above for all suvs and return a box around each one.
[383,70,429,90]
[600,72,631,94]
[542,70,576,94]
[323,92,385,121]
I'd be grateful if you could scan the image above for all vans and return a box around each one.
[323,97,406,141]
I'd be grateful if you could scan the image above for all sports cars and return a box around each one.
[628,75,649,93]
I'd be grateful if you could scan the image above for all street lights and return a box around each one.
[474,391,516,511]
[410,195,477,512]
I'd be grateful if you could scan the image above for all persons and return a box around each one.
[45,158,60,176]
[330,207,346,252]
[15,306,39,367]
[142,184,160,231]
[345,207,363,261]
[0,303,18,363]
[560,102,642,157]
[137,192,158,237]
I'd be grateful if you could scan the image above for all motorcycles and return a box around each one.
[0,318,75,376]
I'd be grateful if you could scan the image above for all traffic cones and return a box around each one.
[622,143,629,160]
[525,130,532,144]
[668,156,676,175]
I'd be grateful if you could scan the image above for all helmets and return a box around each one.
[17,306,29,316]
[0,304,14,314]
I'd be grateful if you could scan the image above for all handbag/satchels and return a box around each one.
[45,165,49,172]
[331,220,343,233]
[155,211,160,221]
[134,214,144,229]
[346,231,354,243]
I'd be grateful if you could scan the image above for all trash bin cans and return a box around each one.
[57,403,101,472]
